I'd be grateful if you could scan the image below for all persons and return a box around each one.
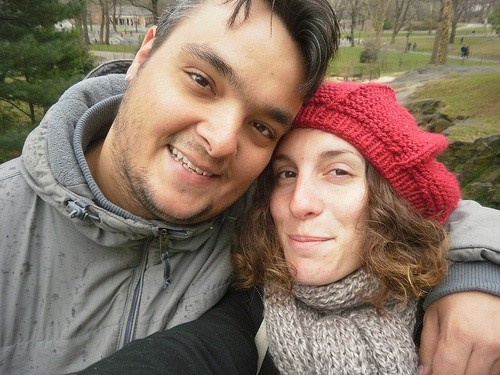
[0,0,500,375]
[61,81,462,375]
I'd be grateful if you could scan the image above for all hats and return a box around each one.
[292,82,460,225]
